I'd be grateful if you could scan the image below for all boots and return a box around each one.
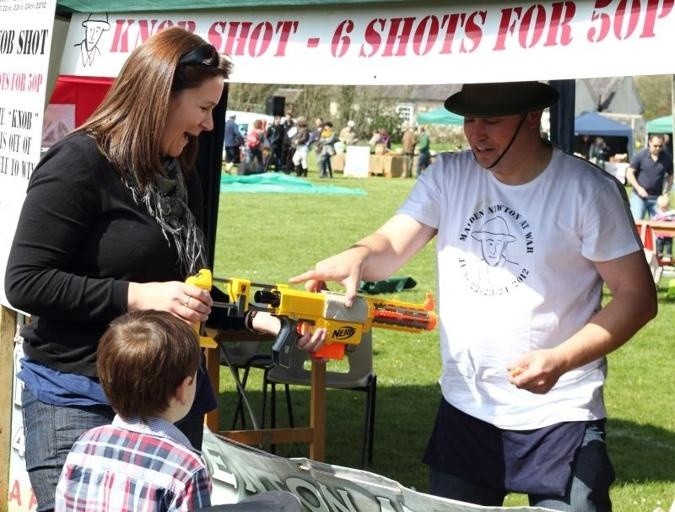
[295,165,308,177]
[225,162,235,175]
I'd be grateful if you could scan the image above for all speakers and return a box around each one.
[266,96,285,116]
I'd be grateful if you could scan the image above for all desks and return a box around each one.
[328,153,412,178]
[632,217,675,244]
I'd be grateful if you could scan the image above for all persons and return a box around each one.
[648,193,674,261]
[73,13,111,67]
[5,28,325,512]
[540,129,609,170]
[53,309,212,512]
[289,81,658,510]
[627,135,675,223]
[223,113,431,182]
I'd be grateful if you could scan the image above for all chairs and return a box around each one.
[632,223,675,285]
[265,319,378,468]
[215,315,298,458]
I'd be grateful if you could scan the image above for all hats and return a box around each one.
[443,81,561,118]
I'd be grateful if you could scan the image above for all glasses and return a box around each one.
[178,42,220,68]
[653,144,663,148]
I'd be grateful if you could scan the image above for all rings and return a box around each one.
[186,296,190,308]
